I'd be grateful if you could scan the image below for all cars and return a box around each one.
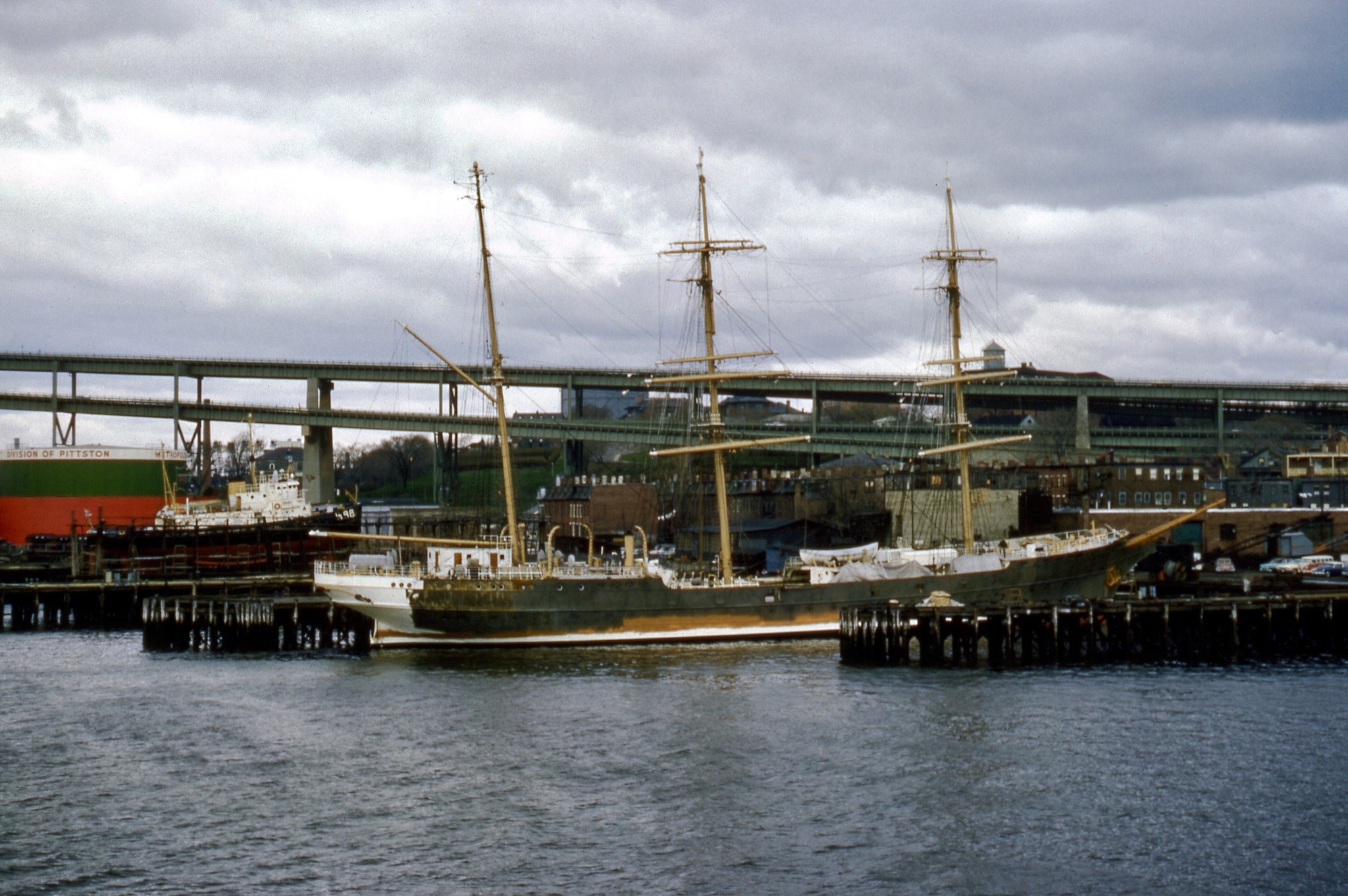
[1259,556,1348,578]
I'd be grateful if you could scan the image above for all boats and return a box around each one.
[26,411,367,577]
[0,538,77,583]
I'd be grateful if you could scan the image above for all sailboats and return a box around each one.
[296,163,1227,663]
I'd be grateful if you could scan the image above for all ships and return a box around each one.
[0,440,189,544]
